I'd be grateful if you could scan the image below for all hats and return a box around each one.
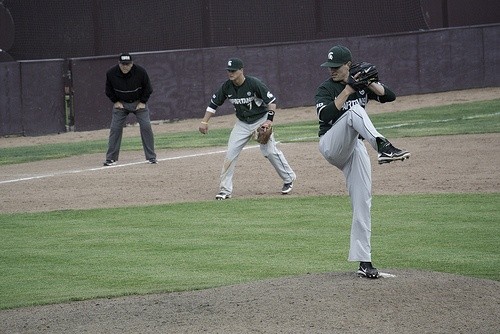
[226,58,244,70]
[320,45,352,68]
[119,53,132,64]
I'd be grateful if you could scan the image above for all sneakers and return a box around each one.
[216,192,231,200]
[149,159,159,165]
[356,265,379,279]
[281,173,297,193]
[103,160,117,165]
[377,149,411,165]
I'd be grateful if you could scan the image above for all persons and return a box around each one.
[199,58,297,200]
[103,53,158,166]
[313,44,412,278]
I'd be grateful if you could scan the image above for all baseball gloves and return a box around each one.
[347,59,380,98]
[253,120,273,145]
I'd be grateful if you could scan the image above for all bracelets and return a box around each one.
[201,122,208,125]
